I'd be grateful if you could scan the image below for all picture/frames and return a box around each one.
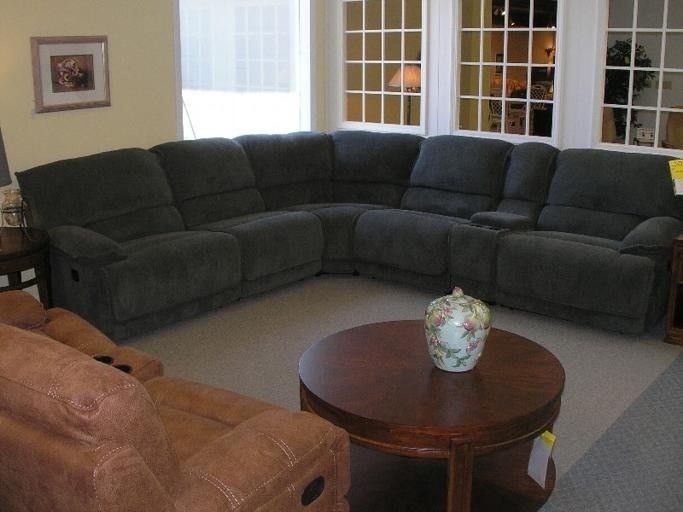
[30,36,111,114]
[494,52,504,74]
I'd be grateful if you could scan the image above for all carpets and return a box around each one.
[116,274,683,481]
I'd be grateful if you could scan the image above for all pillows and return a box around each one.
[0,322,180,486]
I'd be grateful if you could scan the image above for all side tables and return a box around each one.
[0,227,54,310]
[663,233,683,346]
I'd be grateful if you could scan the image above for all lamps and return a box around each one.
[508,19,515,27]
[388,65,421,125]
[498,5,506,15]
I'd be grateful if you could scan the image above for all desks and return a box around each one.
[633,136,655,147]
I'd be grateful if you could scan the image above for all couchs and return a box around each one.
[0,290,352,511]
[15,131,683,344]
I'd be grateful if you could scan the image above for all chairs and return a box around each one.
[489,82,547,136]
[662,106,683,150]
[602,108,625,144]
